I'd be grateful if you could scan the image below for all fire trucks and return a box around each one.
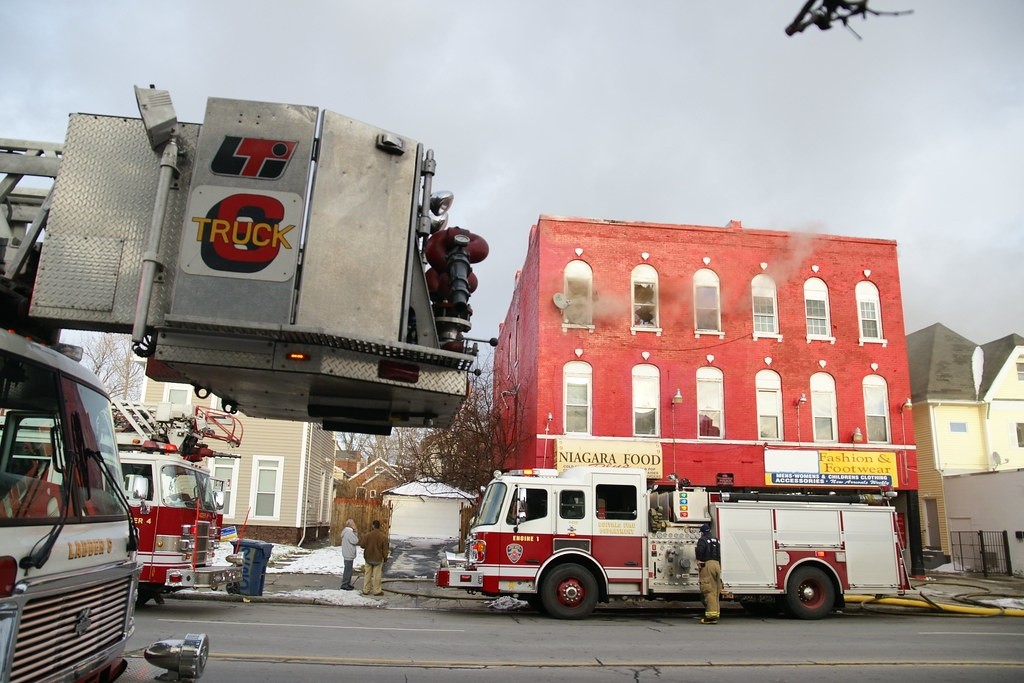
[438,470,912,618]
[0,85,488,683]
[112,402,245,608]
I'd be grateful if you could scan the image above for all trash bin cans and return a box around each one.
[227,538,275,599]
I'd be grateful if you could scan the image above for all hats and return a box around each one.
[698,524,710,531]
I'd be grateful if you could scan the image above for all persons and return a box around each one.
[360,520,388,597]
[340,519,359,591]
[696,525,721,624]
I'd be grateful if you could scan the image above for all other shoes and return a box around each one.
[341,587,352,590]
[701,618,718,624]
[349,584,354,588]
[374,592,384,596]
